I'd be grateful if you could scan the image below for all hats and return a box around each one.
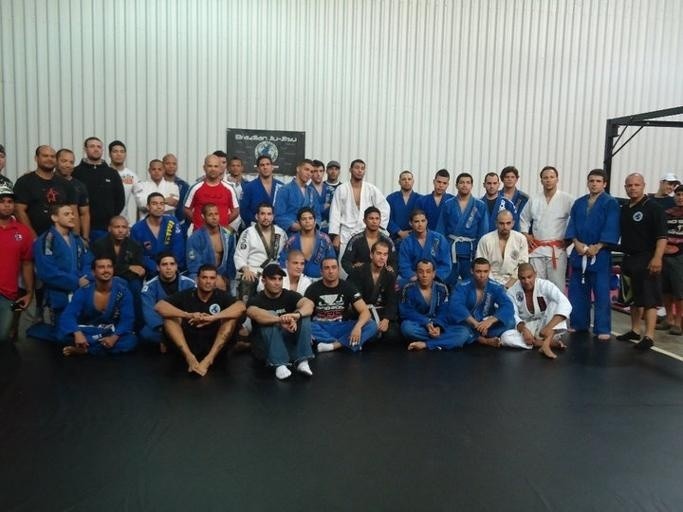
[0,185,15,197]
[660,173,681,186]
[326,161,341,169]
[262,264,287,278]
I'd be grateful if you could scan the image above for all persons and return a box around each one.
[7,138,622,358]
[620,174,668,351]
[247,263,316,379]
[646,173,683,337]
[154,265,247,377]
